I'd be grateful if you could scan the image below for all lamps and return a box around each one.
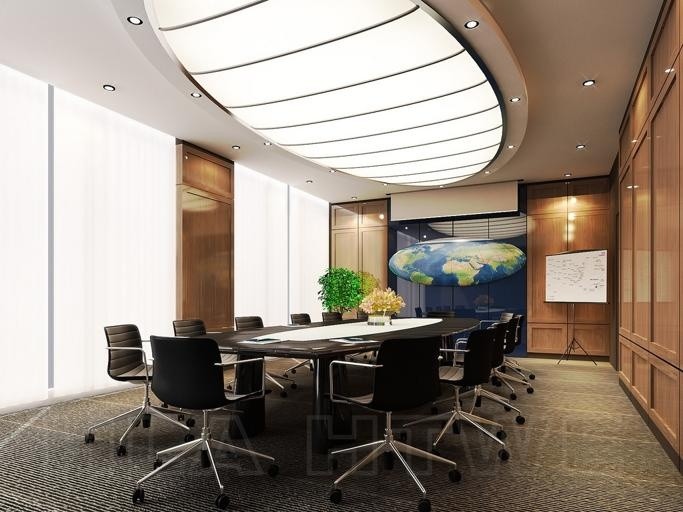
[140,1,507,187]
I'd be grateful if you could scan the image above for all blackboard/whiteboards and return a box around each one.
[543,247,609,305]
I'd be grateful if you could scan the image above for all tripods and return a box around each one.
[557,304,598,367]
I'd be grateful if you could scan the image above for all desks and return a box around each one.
[202,315,481,447]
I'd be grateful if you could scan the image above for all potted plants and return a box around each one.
[357,287,407,326]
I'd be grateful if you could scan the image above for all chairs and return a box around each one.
[400,329,510,461]
[228,316,297,398]
[283,308,538,424]
[84,323,197,456]
[160,319,231,408]
[319,336,461,510]
[131,336,279,510]
